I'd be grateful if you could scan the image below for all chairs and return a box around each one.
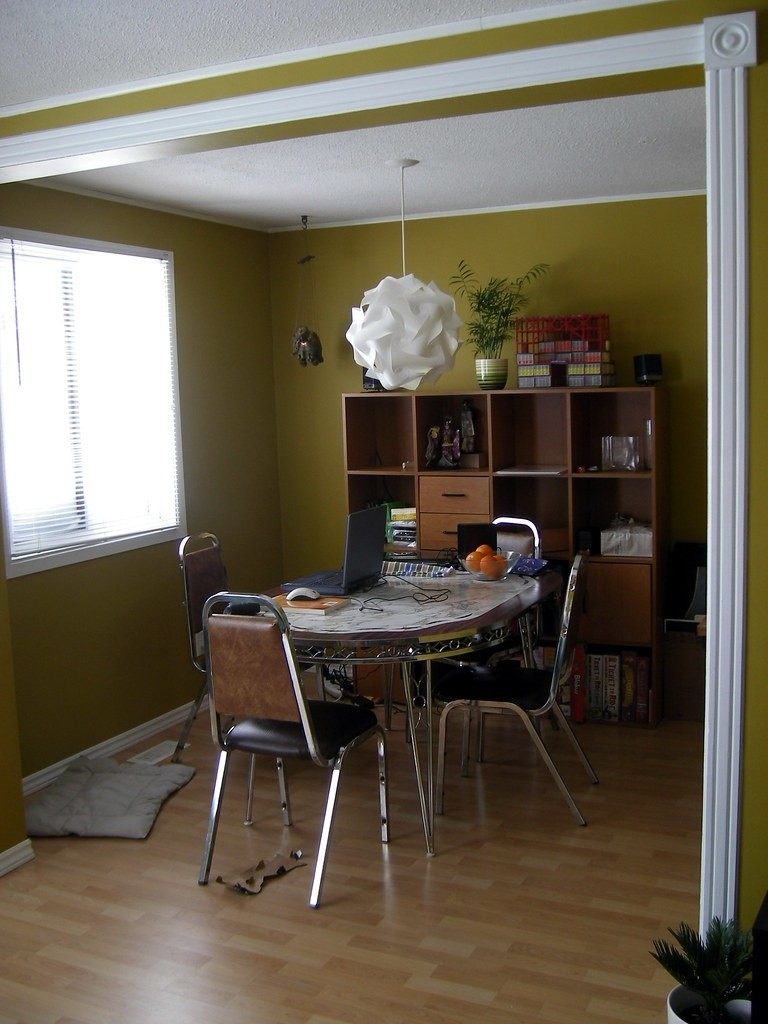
[435,551,599,828]
[477,515,559,763]
[171,531,326,764]
[198,591,392,909]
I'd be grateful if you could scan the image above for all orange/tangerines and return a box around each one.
[466,544,507,577]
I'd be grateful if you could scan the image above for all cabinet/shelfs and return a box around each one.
[341,386,671,732]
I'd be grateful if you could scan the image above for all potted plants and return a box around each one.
[449,258,551,390]
[648,915,755,1024]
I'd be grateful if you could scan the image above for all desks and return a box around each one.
[220,557,563,857]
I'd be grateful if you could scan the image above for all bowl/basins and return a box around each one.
[456,550,519,582]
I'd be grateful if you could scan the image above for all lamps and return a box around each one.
[345,157,464,391]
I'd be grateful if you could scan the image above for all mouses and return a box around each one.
[285,587,321,602]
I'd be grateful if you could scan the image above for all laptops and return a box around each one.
[280,504,389,596]
